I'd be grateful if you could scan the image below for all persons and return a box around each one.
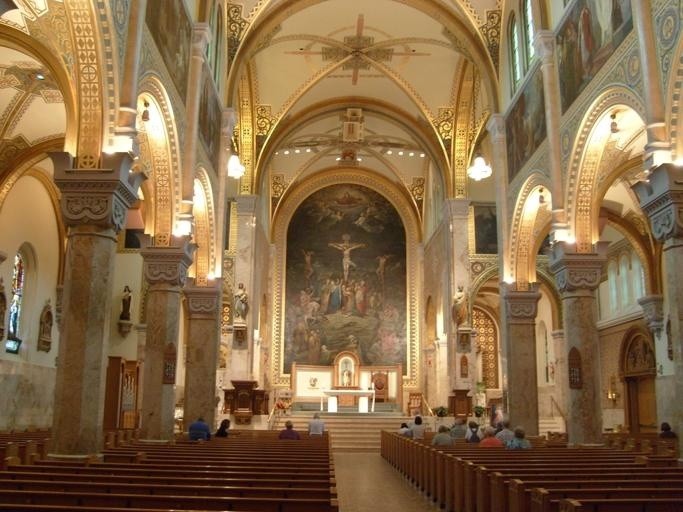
[495,422,513,441]
[292,189,398,362]
[493,421,503,435]
[503,426,531,450]
[555,1,598,108]
[397,423,408,435]
[306,412,324,436]
[277,420,303,440]
[658,422,677,438]
[232,281,248,321]
[463,421,482,441]
[480,426,501,446]
[213,418,230,437]
[430,424,456,447]
[449,418,467,438]
[188,413,210,441]
[409,415,425,440]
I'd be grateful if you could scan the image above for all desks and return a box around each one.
[323,390,374,413]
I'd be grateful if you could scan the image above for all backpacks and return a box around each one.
[470,428,480,442]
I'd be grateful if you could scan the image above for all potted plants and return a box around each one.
[436,407,448,417]
[473,406,485,417]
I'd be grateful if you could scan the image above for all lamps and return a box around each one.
[226,144,245,182]
[142,102,149,121]
[539,188,543,203]
[466,64,492,182]
[610,114,618,132]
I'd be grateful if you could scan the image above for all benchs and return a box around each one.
[379,429,683,512]
[0,429,339,512]
